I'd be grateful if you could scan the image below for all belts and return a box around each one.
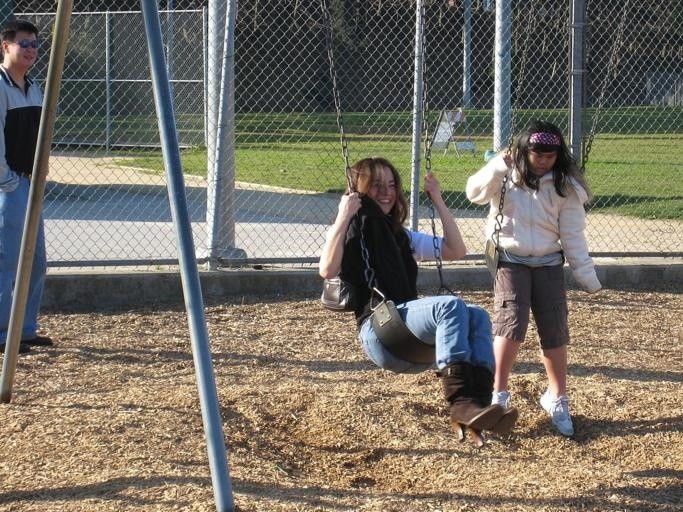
[14,172,32,180]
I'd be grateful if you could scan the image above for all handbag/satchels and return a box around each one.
[320,273,358,313]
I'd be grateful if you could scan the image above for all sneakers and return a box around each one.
[490,390,510,410]
[538,387,574,436]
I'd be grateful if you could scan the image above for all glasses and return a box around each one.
[4,39,38,50]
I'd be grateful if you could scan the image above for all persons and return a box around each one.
[0,16,55,354]
[317,155,519,448]
[463,118,603,438]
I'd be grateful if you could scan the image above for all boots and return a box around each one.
[434,361,505,443]
[467,363,518,450]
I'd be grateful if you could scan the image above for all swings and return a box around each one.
[320,0,456,364]
[486,0,629,278]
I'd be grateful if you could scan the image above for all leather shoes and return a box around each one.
[20,336,53,347]
[0,342,30,353]
